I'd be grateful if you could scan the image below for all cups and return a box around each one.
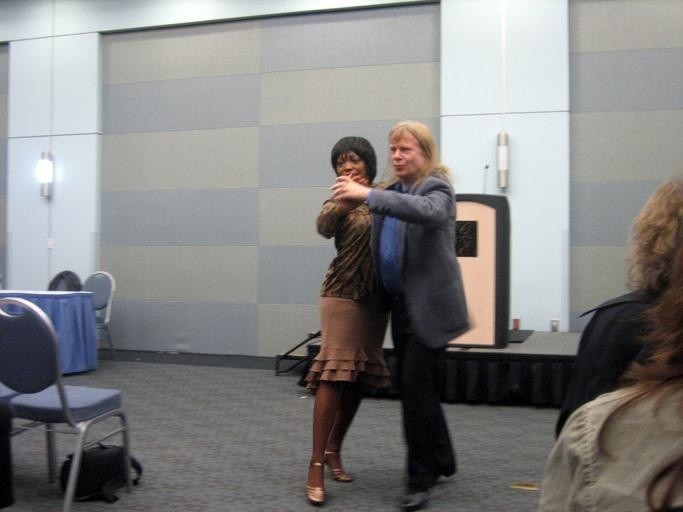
[549,319,561,331]
[512,318,521,330]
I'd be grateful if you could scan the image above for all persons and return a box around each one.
[329,120,478,509]
[538,241,683,511]
[305,137,394,506]
[555,177,683,438]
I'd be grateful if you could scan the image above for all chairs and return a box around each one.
[82,271,115,357]
[0,299,131,512]
[49,271,81,291]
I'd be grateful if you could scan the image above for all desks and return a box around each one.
[0,290,97,375]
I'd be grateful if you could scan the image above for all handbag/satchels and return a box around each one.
[62,443,142,503]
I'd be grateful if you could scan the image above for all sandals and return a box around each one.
[306,462,327,504]
[324,451,352,481]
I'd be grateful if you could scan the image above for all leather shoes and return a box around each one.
[399,487,428,512]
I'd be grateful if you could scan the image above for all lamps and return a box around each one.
[498,132,508,187]
[39,150,51,194]
